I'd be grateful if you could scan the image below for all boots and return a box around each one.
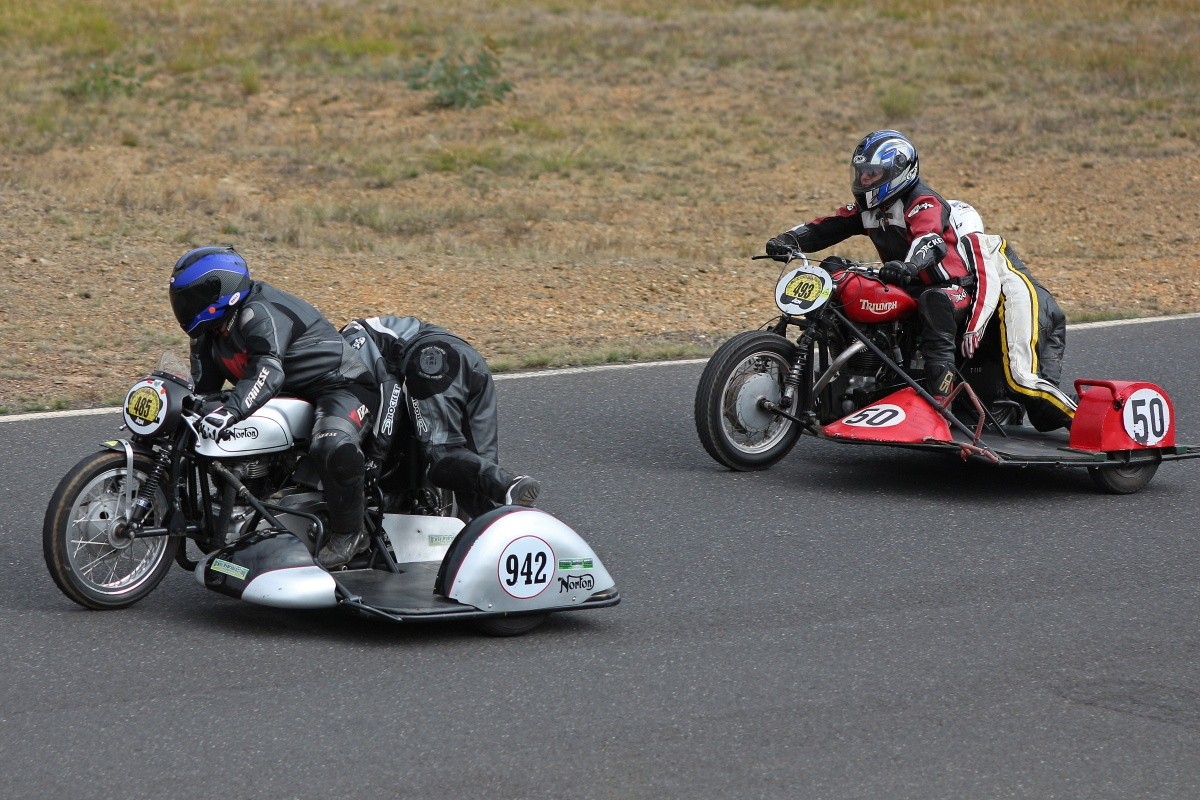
[931,367,955,430]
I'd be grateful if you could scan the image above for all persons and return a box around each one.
[169,247,381,569]
[339,316,540,522]
[766,130,974,429]
[944,197,1081,433]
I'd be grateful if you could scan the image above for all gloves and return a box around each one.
[198,406,235,445]
[766,231,802,264]
[878,260,921,288]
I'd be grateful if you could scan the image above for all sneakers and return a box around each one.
[318,526,371,570]
[487,474,543,510]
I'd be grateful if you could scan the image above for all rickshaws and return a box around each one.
[41,369,621,640]
[693,243,1199,495]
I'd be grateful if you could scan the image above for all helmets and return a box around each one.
[849,129,919,211]
[169,245,254,344]
